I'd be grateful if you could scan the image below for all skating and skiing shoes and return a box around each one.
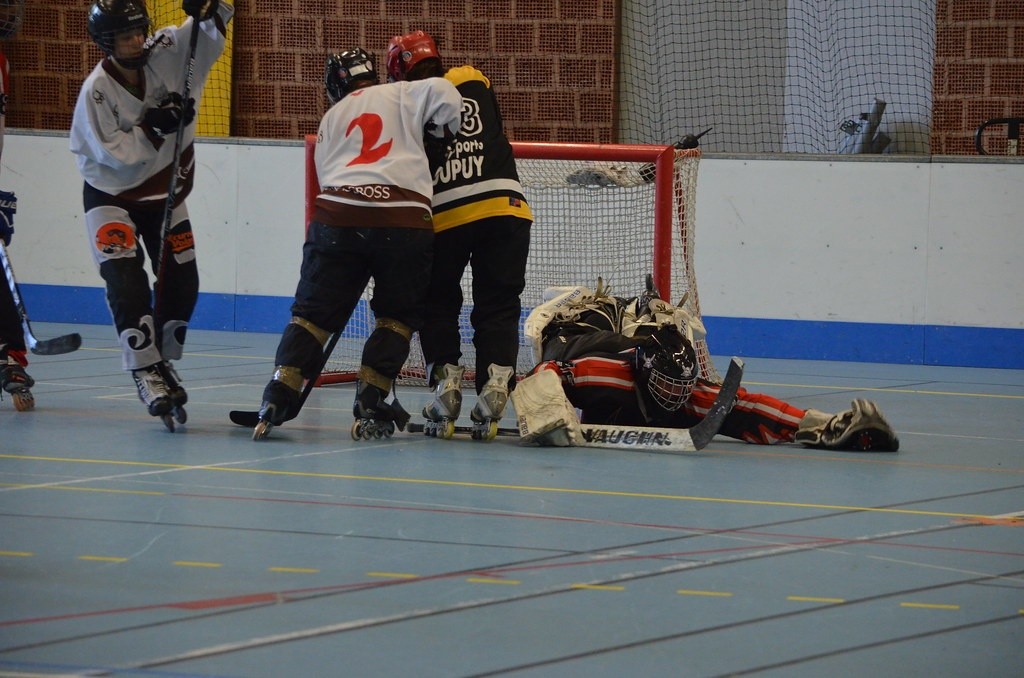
[351,380,397,441]
[470,363,514,443]
[0,343,35,411]
[132,366,176,433]
[156,361,188,424]
[252,380,301,440]
[421,362,465,439]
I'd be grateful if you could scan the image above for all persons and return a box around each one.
[252,48,464,441]
[70,0,237,433]
[507,277,900,452]
[0,51,34,412]
[384,31,535,440]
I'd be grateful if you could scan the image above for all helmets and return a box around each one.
[324,47,378,106]
[633,324,700,416]
[386,30,443,83]
[87,0,165,69]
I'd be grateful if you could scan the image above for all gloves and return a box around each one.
[182,0,219,21]
[424,123,457,186]
[140,92,196,150]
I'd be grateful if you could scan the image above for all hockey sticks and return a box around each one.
[1,237,82,356]
[405,356,745,454]
[152,17,202,346]
